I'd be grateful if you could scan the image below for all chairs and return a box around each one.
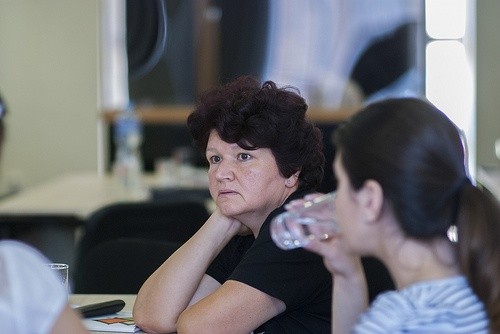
[73,200,210,294]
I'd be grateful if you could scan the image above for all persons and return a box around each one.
[0,92,94,334]
[288,94,500,334]
[130,76,398,334]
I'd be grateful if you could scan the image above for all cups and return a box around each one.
[270,193,341,251]
[47,263,68,292]
